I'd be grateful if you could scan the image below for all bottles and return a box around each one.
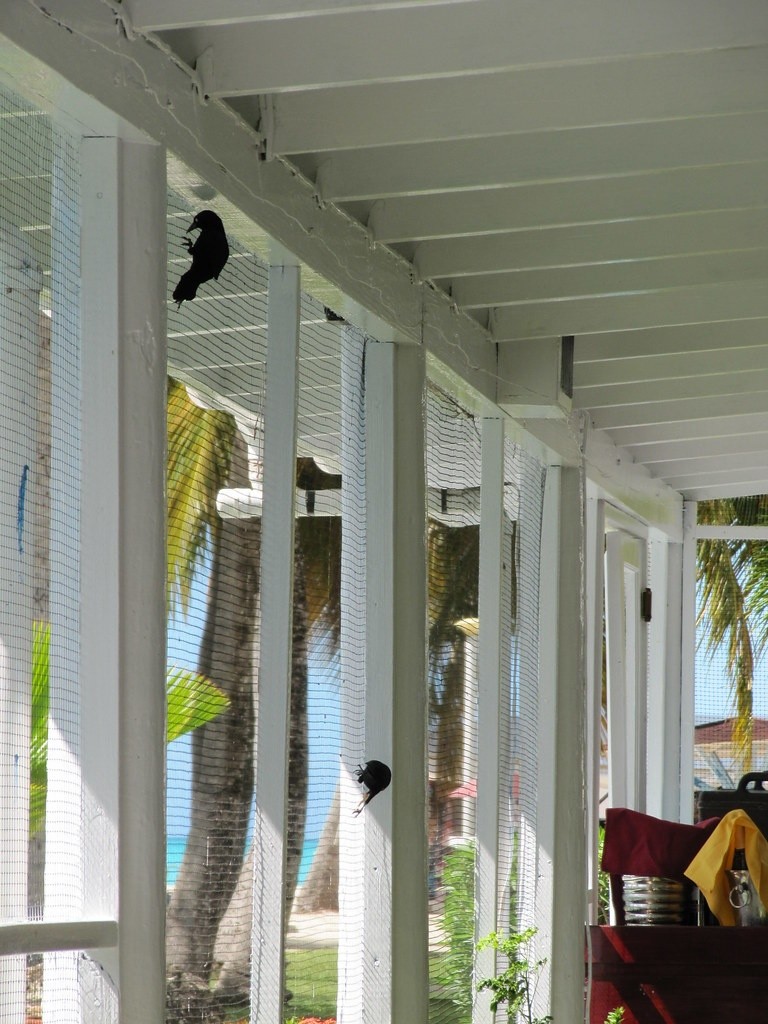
[725,817,766,928]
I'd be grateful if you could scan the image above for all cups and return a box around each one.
[689,874,716,926]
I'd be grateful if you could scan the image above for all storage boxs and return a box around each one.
[698,771,768,869]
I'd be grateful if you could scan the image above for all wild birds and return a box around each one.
[173,208,229,307]
[351,760,391,818]
